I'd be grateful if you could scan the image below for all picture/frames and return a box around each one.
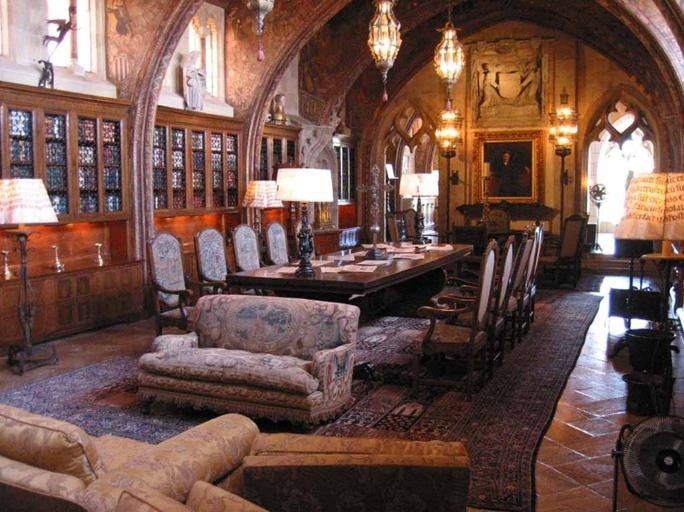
[473,129,544,204]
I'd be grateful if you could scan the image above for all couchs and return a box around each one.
[136,294,361,425]
[0,412,260,512]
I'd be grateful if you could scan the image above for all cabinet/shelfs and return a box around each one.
[0,81,145,355]
[260,123,303,180]
[153,104,241,313]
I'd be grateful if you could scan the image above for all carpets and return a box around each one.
[0,270,613,512]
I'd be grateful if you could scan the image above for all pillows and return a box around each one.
[0,402,109,482]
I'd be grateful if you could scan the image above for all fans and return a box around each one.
[590,183,608,251]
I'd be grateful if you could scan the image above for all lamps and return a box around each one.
[0,178,60,374]
[433,0,466,101]
[242,168,335,276]
[245,0,275,59]
[403,174,439,244]
[613,172,684,418]
[549,85,578,250]
[435,89,461,243]
[366,0,403,102]
